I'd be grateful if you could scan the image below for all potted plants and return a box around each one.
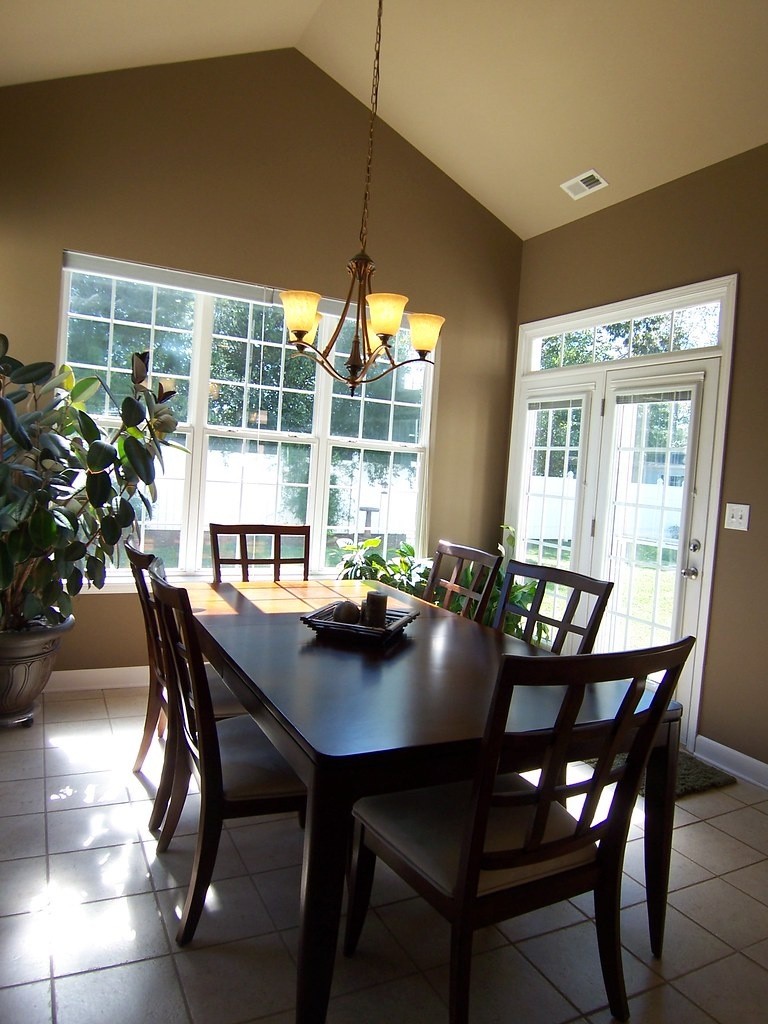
[0,330,193,730]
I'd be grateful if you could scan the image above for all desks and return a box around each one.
[170,574,685,1024]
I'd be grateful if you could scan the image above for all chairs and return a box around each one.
[419,539,615,812]
[149,556,348,948]
[207,521,313,585]
[124,534,247,834]
[338,636,696,1024]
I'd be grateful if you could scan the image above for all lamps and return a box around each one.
[277,0,447,397]
